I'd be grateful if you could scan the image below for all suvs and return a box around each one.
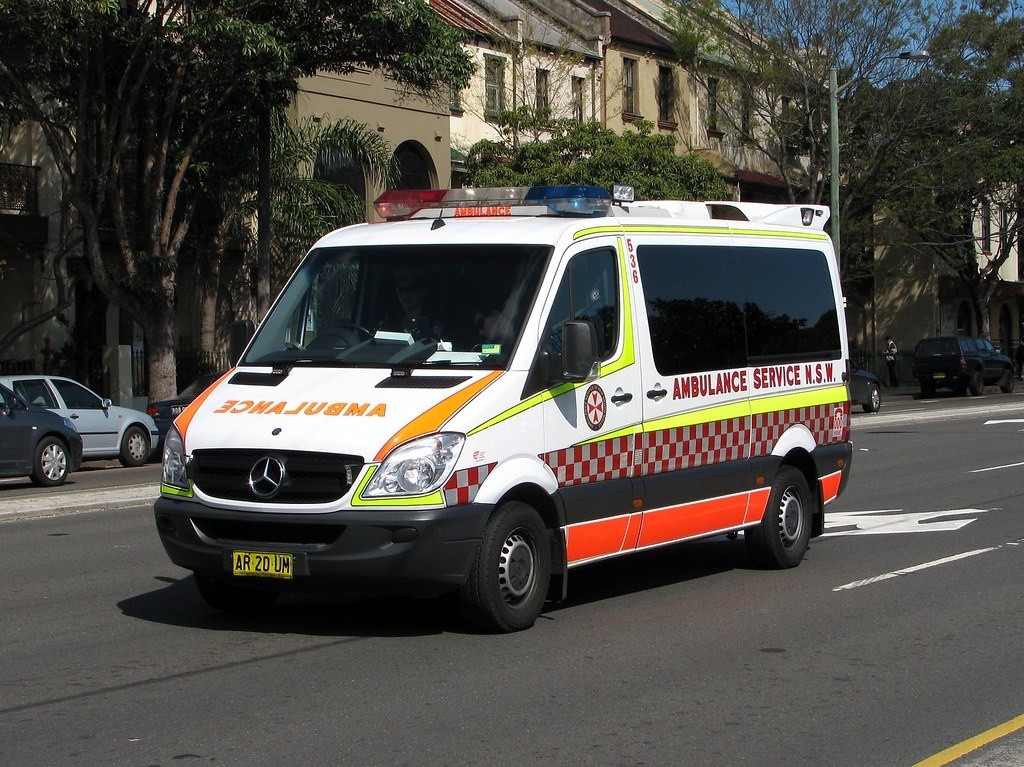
[913,337,1017,398]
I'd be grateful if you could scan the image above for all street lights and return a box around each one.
[830,50,931,275]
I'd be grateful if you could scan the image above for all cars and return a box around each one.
[0,375,159,470]
[0,384,82,488]
[150,375,220,451]
[849,358,881,413]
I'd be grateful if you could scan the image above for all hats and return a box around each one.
[883,336,894,340]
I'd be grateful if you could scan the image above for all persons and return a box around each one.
[472,300,524,346]
[884,336,901,387]
[361,264,457,350]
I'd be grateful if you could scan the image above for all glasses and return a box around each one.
[396,284,426,294]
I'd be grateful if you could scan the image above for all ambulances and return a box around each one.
[156,183,854,633]
